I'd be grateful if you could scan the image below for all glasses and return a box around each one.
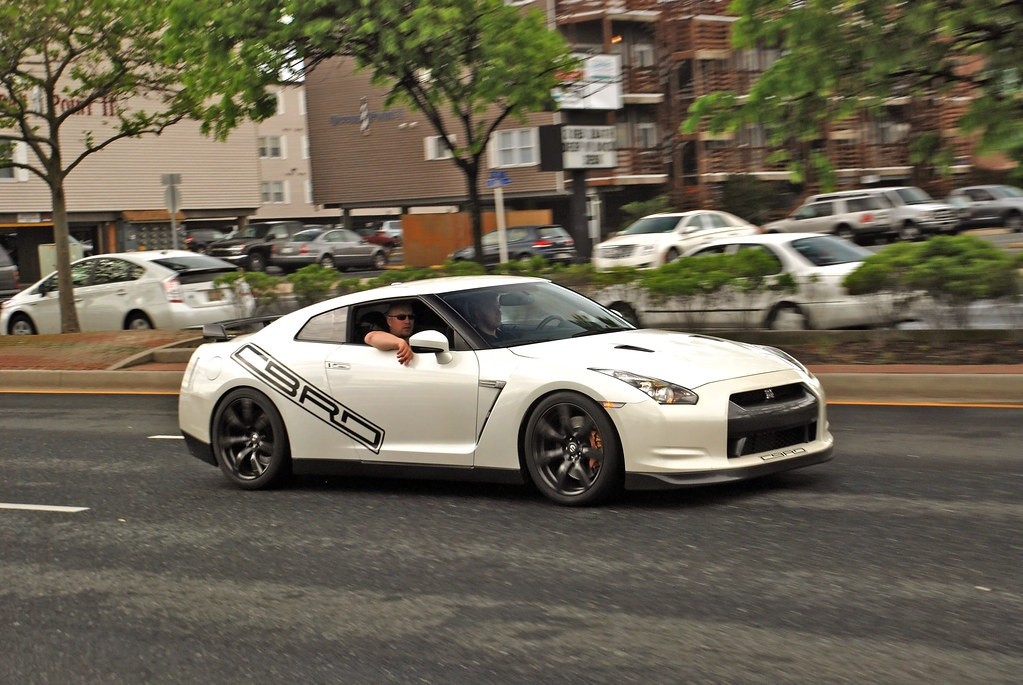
[389,314,416,321]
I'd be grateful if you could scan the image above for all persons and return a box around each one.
[364,301,414,365]
[471,293,512,341]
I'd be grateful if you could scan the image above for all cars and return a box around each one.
[356,229,402,247]
[941,185,1023,237]
[0,251,257,337]
[271,227,389,273]
[593,210,760,274]
[178,272,833,505]
[848,186,961,239]
[583,232,922,330]
[445,224,575,273]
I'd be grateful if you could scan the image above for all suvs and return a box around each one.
[759,193,892,247]
[207,221,305,271]
